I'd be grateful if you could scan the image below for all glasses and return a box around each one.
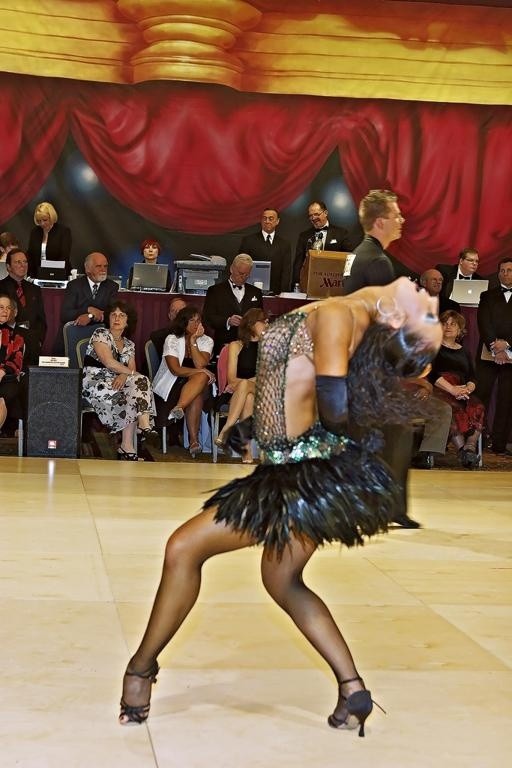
[237,268,250,278]
[308,211,324,219]
[464,257,480,265]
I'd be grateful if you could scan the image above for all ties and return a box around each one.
[266,235,272,247]
[17,288,26,307]
[93,285,98,295]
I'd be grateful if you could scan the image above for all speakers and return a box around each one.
[26,366,82,458]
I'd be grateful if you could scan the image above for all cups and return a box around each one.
[254,282,263,289]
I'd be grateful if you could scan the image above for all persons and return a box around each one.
[55,251,158,462]
[419,268,460,320]
[475,258,512,456]
[291,198,350,292]
[0,232,48,437]
[342,189,420,529]
[434,248,483,298]
[26,202,74,282]
[203,255,266,463]
[427,309,485,470]
[119,276,445,738]
[128,239,173,293]
[398,356,453,470]
[210,207,291,296]
[152,298,216,457]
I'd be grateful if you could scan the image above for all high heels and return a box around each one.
[118,662,159,723]
[189,442,202,459]
[328,677,373,737]
[137,428,158,441]
[116,447,144,461]
[168,406,185,421]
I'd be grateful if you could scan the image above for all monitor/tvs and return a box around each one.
[245,260,272,294]
[130,263,169,291]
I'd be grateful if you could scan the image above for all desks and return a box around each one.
[38,285,500,433]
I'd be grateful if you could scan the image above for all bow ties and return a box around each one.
[459,273,470,280]
[233,285,242,290]
[500,287,512,292]
[315,226,328,232]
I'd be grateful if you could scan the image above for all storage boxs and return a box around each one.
[298,249,354,298]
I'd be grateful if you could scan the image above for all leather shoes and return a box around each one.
[395,516,418,528]
[241,455,253,464]
[416,453,439,469]
[460,446,480,469]
[215,438,226,448]
[492,446,512,456]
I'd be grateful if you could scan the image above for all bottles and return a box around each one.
[294,283,301,293]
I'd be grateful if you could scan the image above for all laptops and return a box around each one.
[453,279,489,304]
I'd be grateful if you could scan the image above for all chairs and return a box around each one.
[63,321,106,369]
[75,338,98,456]
[15,343,27,456]
[144,337,212,454]
[212,411,267,463]
[429,429,485,468]
[405,417,429,469]
